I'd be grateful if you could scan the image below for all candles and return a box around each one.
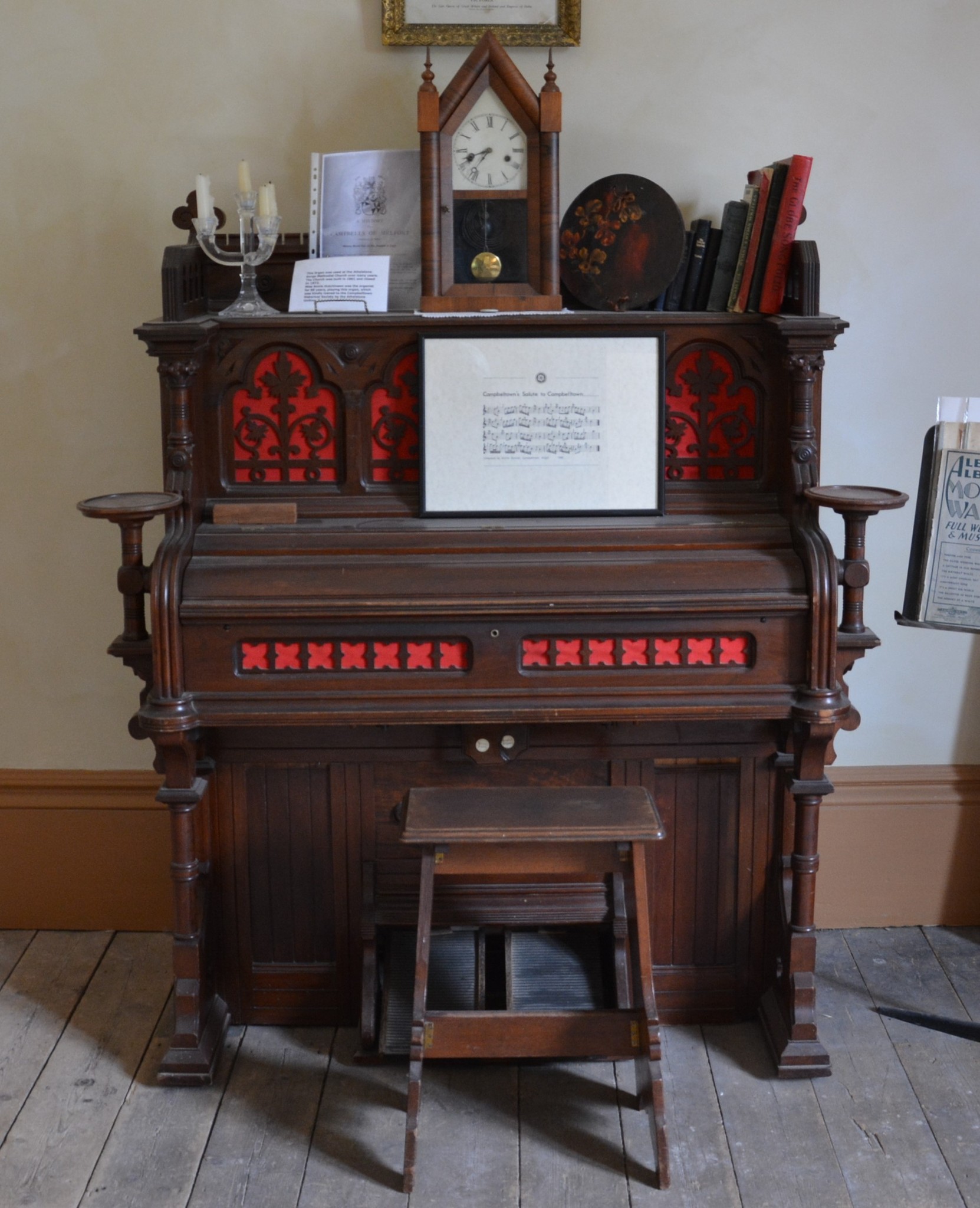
[239,158,253,194]
[259,184,271,216]
[265,180,278,215]
[196,172,211,218]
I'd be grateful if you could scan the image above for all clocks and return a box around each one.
[416,27,565,312]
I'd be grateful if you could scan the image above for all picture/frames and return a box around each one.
[414,330,667,521]
[382,0,581,48]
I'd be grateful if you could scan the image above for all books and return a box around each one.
[653,155,814,312]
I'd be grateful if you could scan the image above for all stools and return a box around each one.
[400,784,670,1193]
